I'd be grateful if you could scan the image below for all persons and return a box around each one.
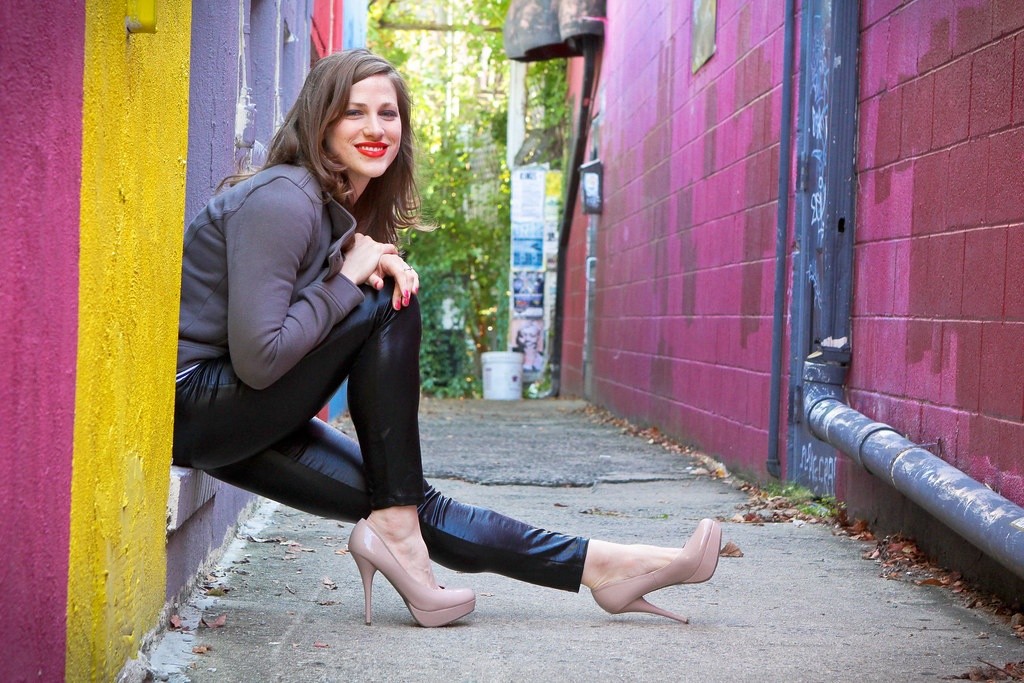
[513,321,544,372]
[441,272,466,378]
[171,49,722,628]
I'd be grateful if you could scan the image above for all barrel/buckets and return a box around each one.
[480,350,522,403]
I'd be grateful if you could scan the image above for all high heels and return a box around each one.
[591,518,722,624]
[348,518,475,628]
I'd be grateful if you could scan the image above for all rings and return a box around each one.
[404,265,413,271]
